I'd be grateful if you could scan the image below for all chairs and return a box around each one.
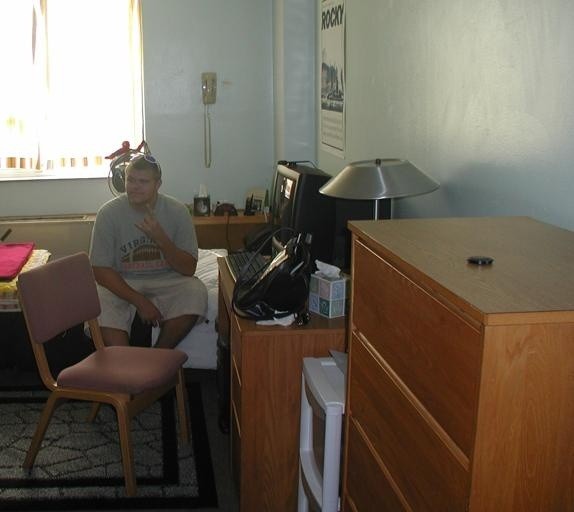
[14,251,191,495]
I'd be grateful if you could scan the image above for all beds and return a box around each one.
[0,241,231,388]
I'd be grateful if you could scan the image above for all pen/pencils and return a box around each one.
[250,193,254,209]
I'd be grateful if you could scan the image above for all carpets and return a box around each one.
[0,372,220,511]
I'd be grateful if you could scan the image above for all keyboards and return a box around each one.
[223,251,269,288]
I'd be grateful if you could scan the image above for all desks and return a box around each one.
[215,255,349,511]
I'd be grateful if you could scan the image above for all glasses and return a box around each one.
[131,152,157,164]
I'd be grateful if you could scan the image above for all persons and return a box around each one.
[84,155,208,348]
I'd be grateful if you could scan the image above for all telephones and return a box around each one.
[201,73,216,104]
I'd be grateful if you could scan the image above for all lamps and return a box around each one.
[319,156,441,222]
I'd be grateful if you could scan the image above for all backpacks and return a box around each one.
[232,228,308,320]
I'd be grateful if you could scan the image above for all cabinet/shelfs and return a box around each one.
[297,352,349,512]
[342,214,573,511]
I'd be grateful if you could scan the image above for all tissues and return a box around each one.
[193,184,211,216]
[308,257,351,318]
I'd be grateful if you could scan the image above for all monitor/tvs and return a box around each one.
[270,164,392,281]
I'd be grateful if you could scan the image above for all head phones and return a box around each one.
[110,156,135,193]
